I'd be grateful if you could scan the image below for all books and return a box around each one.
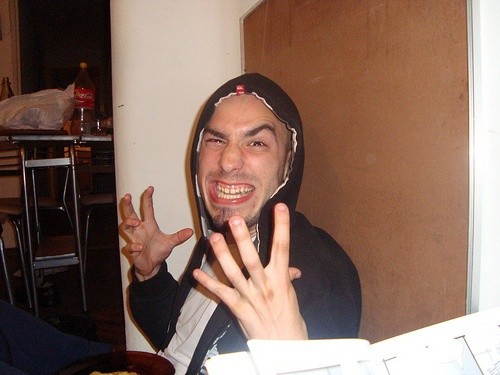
[0,149,22,170]
[64,141,92,163]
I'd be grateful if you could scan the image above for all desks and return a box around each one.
[0,131,115,287]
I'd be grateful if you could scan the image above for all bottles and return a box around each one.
[0,76,15,102]
[71,62,96,136]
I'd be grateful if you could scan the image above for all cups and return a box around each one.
[91,112,107,136]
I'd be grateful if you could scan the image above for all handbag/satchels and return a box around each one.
[0,82,76,130]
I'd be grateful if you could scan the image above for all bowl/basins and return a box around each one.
[56,351,175,375]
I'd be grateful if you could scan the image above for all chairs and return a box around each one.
[0,141,116,317]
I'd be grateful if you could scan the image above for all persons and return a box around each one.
[0,73,362,375]
